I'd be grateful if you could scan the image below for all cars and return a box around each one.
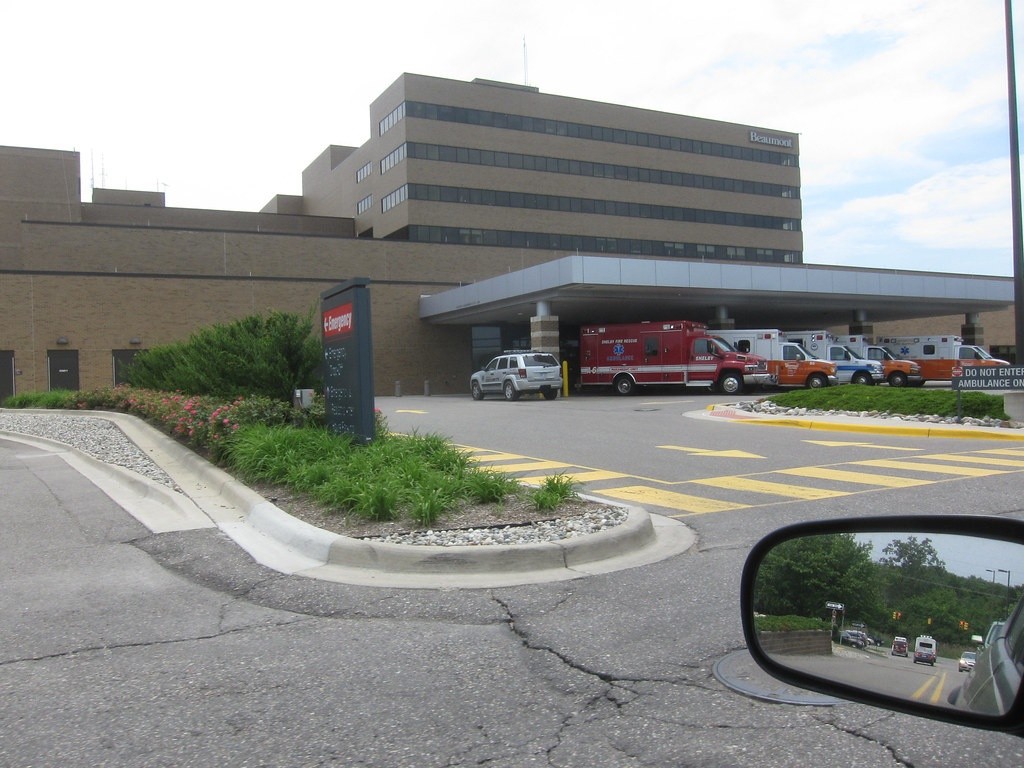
[959,652,975,672]
[947,567,1024,715]
[913,647,934,665]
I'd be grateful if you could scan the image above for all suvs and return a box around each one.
[972,621,1006,663]
[469,349,563,400]
[837,629,885,649]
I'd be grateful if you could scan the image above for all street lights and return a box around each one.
[998,569,1011,625]
[985,569,996,621]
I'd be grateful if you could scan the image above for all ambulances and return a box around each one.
[833,334,923,387]
[580,320,769,399]
[783,330,883,385]
[876,334,1010,381]
[706,328,837,389]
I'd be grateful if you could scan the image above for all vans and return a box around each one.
[915,635,938,662]
[891,637,909,657]
